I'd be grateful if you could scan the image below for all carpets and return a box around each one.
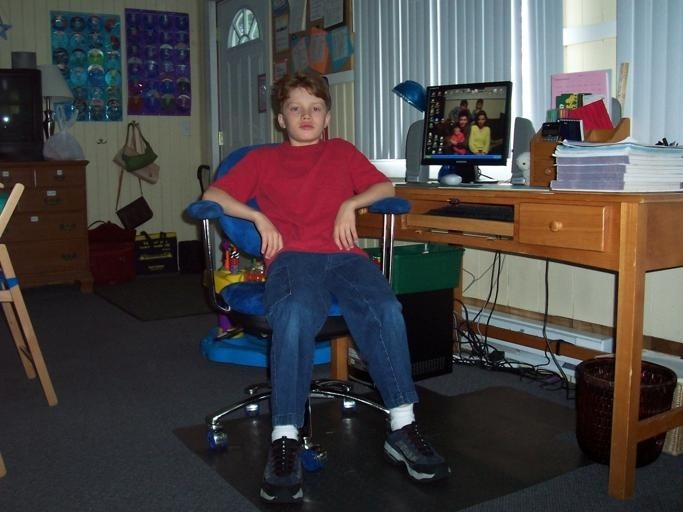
[93,275,213,322]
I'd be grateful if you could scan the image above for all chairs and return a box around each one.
[188,142,413,472]
[0,181,60,410]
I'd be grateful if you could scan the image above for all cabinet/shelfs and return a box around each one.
[0,158,92,293]
[529,118,632,187]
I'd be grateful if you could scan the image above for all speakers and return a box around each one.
[406,120,429,183]
[512,117,536,186]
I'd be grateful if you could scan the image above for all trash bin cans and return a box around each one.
[574,356,678,469]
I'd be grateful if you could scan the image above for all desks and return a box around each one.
[330,180,680,504]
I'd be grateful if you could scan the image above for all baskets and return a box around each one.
[576,352,682,468]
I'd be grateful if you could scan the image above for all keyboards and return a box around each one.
[423,206,514,224]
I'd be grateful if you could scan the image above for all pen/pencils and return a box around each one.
[655,138,679,147]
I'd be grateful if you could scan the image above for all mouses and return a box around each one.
[439,173,462,185]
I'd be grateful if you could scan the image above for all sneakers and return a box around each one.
[258,435,305,506]
[383,421,454,484]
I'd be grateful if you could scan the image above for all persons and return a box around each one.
[201,70,451,503]
[437,94,492,157]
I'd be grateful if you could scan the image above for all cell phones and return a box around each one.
[512,177,525,185]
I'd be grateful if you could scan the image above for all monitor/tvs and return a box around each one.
[0,68,44,162]
[421,81,511,183]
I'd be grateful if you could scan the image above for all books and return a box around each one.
[551,137,683,193]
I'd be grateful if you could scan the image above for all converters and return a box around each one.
[489,350,505,361]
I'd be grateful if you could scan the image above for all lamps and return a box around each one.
[39,64,76,140]
[390,80,455,181]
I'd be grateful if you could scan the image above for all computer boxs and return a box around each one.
[347,289,454,389]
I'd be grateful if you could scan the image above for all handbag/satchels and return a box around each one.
[113,121,160,184]
[115,196,154,232]
